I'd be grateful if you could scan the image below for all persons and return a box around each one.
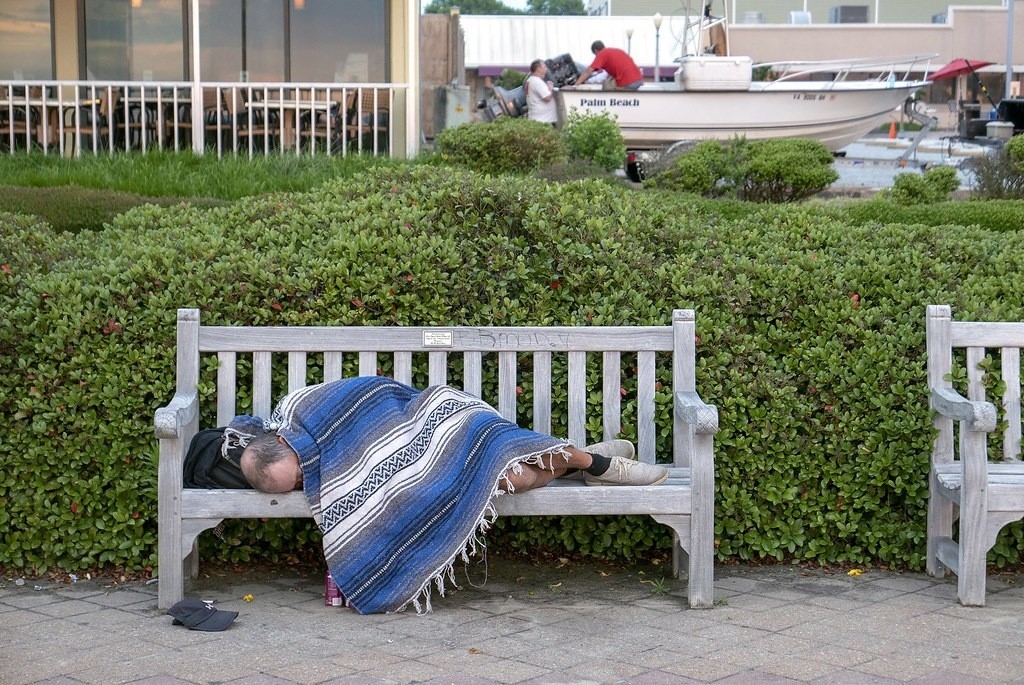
[239,376,671,497]
[572,40,643,91]
[524,59,559,129]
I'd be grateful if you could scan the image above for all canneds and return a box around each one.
[325,570,354,609]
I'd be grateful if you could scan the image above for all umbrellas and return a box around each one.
[926,59,1000,100]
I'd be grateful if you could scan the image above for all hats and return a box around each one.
[165,597,240,632]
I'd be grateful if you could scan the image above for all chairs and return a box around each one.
[0,86,376,158]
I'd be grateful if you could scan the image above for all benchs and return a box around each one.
[155,304,725,620]
[923,301,1024,605]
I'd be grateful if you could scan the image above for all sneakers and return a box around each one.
[584,455,670,486]
[555,439,635,479]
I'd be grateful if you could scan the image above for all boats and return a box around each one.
[545,2,940,177]
